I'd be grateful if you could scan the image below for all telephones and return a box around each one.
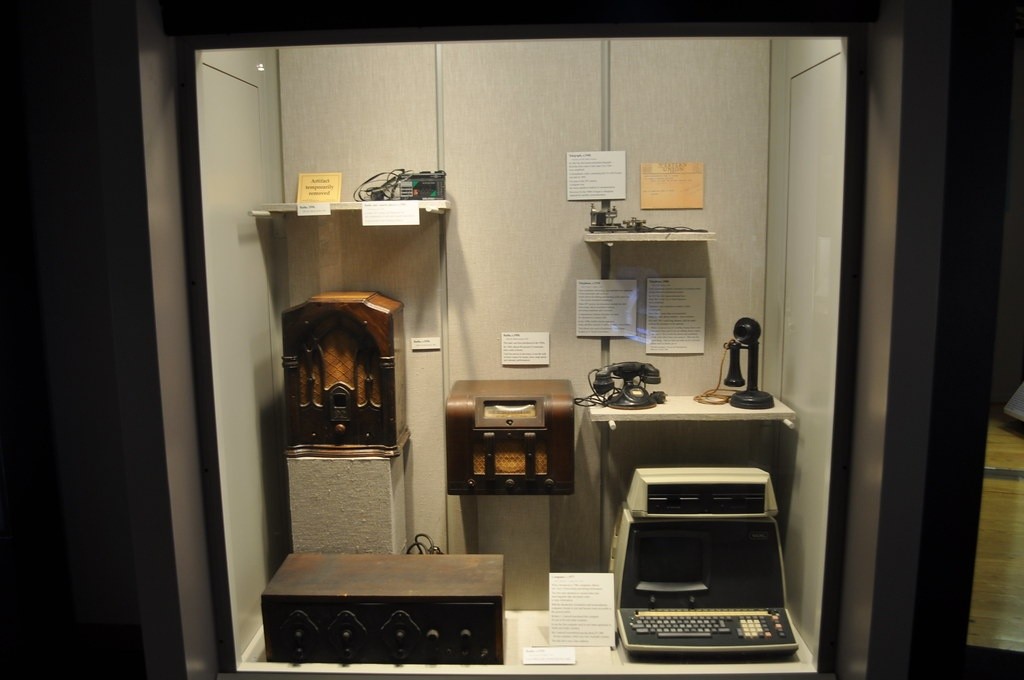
[590,360,662,410]
